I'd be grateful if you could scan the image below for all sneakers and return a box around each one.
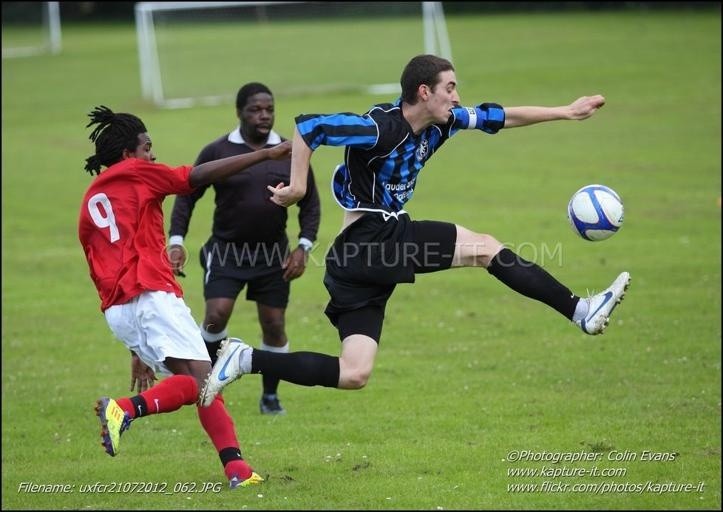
[258,395,288,416]
[228,471,270,490]
[575,270,631,335]
[199,337,250,408]
[94,398,133,457]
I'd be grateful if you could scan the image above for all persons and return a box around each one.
[192,53,634,409]
[165,80,322,421]
[75,100,296,492]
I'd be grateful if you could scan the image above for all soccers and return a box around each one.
[567,184,623,240]
